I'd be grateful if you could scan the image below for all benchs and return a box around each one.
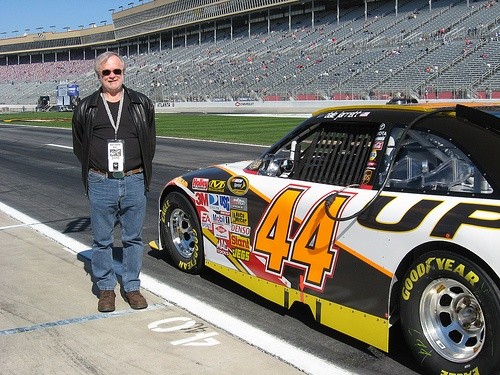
[0,0,500,104]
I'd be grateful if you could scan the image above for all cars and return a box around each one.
[156,99,171,107]
[385,97,419,106]
[148,100,500,375]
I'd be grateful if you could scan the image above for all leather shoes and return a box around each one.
[120,289,148,309]
[98,290,115,311]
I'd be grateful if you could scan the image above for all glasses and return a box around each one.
[101,69,123,75]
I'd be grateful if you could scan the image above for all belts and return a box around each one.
[89,166,143,179]
